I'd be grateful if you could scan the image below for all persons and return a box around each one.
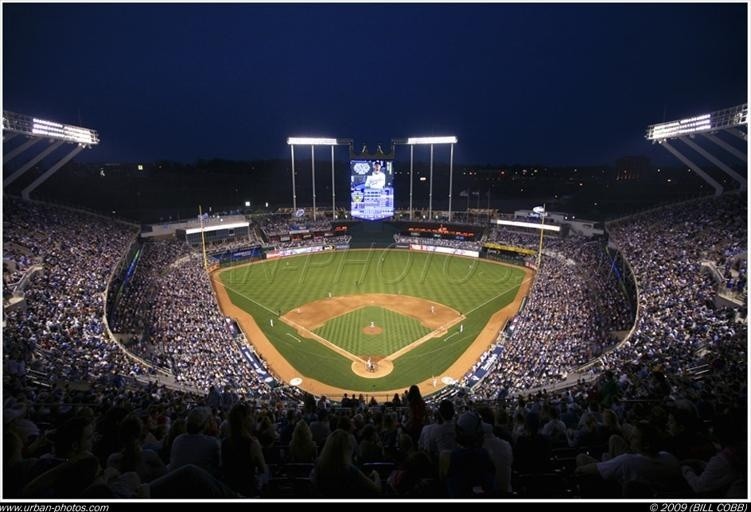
[3,193,749,498]
[363,161,386,191]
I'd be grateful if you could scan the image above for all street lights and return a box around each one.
[288,136,338,221]
[408,136,457,221]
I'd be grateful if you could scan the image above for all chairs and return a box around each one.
[3,439,746,498]
